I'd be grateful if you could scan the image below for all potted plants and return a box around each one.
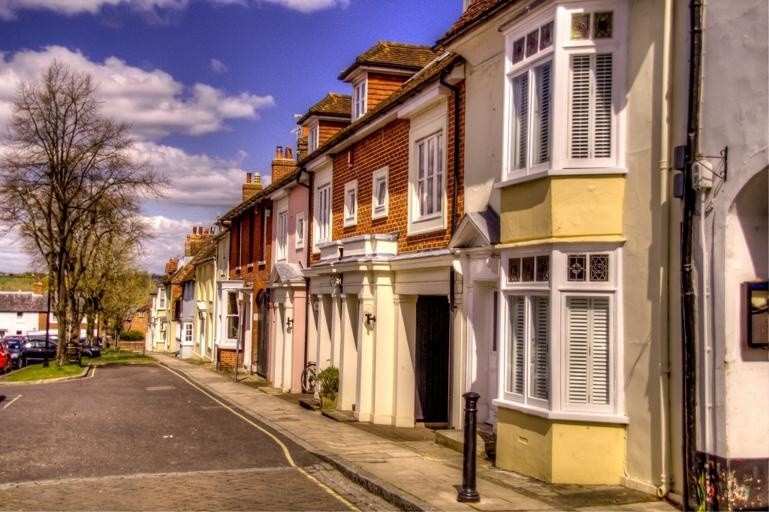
[313,367,341,410]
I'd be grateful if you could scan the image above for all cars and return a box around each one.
[1,335,101,373]
